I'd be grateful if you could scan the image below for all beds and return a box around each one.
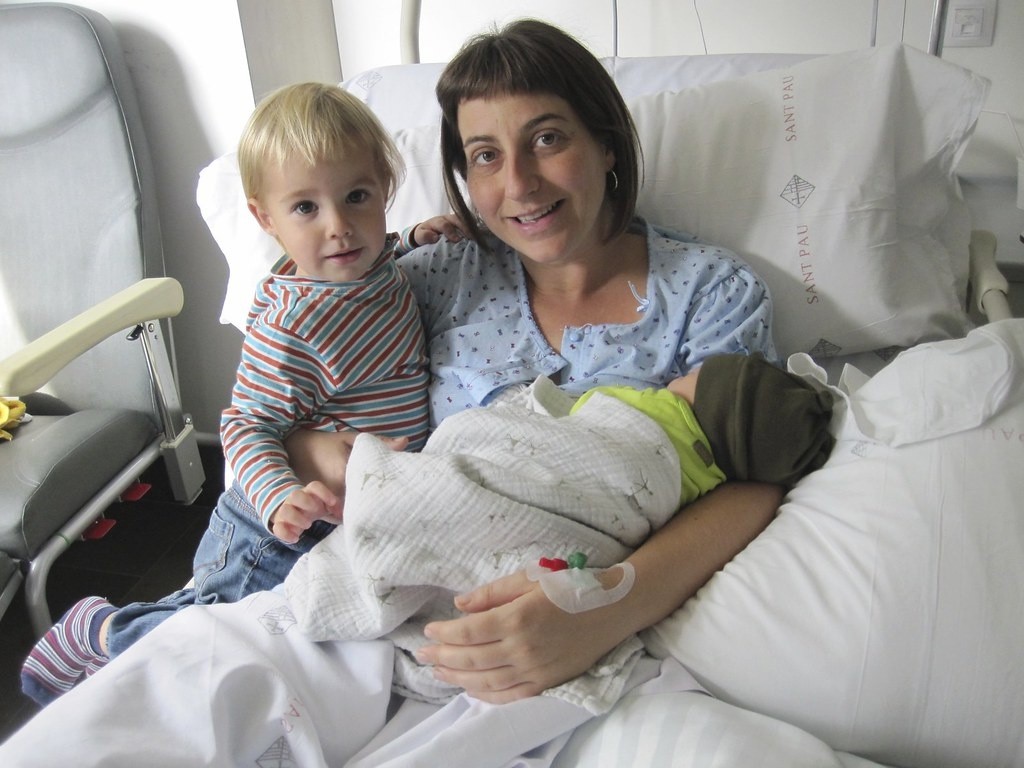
[399,0,1016,388]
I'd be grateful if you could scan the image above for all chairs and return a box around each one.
[0,0,206,643]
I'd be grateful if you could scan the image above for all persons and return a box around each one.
[281,18,780,688]
[279,351,837,642]
[20,80,471,706]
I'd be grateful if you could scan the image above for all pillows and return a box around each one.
[197,40,990,358]
[641,313,1024,768]
